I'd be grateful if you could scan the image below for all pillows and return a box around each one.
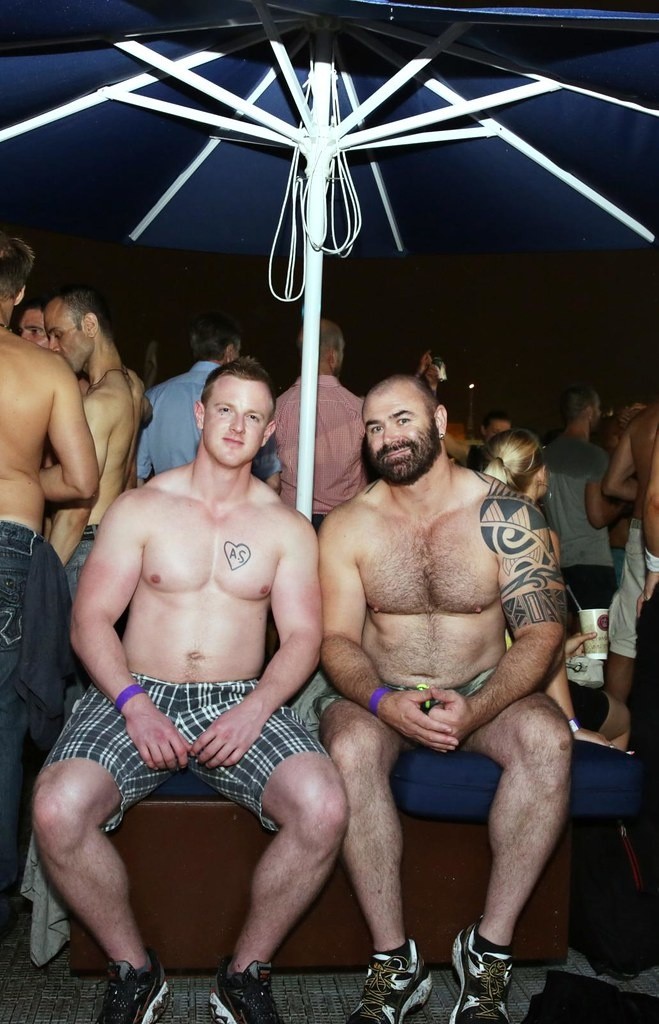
[387,741,646,821]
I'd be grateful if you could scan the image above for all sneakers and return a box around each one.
[348,939,431,1024]
[449,916,516,1024]
[94,948,170,1024]
[208,955,282,1024]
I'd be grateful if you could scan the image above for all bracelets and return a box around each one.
[566,717,582,732]
[415,369,423,377]
[367,685,393,718]
[114,683,146,713]
[432,389,437,397]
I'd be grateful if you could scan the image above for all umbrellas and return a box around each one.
[0,0,659,531]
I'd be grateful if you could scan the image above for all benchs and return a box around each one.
[61,802,571,974]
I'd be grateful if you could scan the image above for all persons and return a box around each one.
[137,316,282,497]
[541,382,628,635]
[425,360,513,472]
[477,429,633,755]
[588,397,659,985]
[409,348,434,377]
[18,300,93,549]
[0,231,101,936]
[30,356,352,1024]
[42,282,154,734]
[317,372,575,1024]
[271,319,374,534]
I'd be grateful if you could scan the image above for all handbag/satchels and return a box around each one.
[569,814,659,982]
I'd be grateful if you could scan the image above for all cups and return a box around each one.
[579,608,610,660]
